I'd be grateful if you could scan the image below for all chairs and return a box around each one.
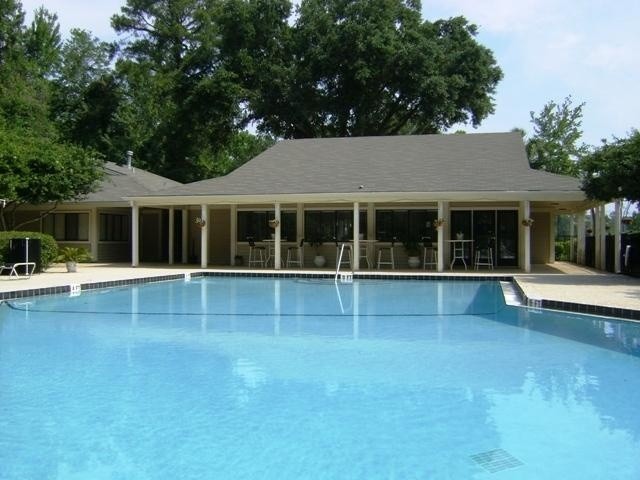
[475,238,495,273]
[286,239,304,269]
[422,237,438,271]
[335,237,351,269]
[377,239,395,270]
[0,261,36,281]
[246,236,267,268]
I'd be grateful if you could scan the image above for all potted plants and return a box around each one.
[192,217,205,229]
[269,219,279,227]
[434,218,449,232]
[523,219,534,227]
[402,235,421,268]
[308,231,327,268]
[54,246,94,273]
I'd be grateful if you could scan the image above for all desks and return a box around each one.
[263,239,287,269]
[349,239,379,270]
[446,240,474,272]
